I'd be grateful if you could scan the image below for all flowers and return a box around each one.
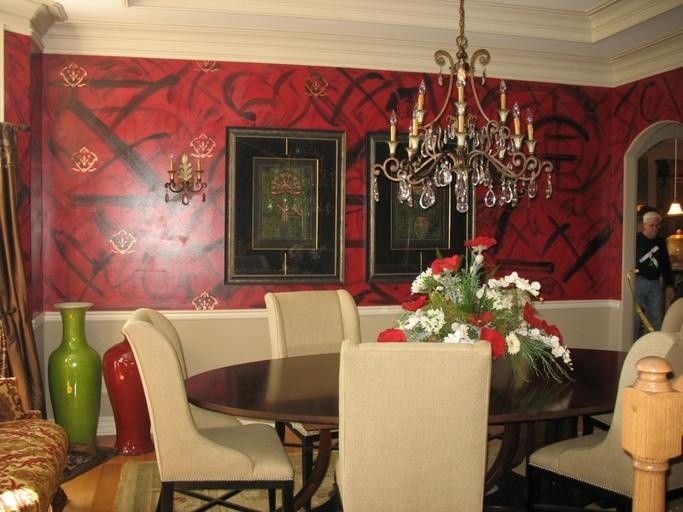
[378,237,577,384]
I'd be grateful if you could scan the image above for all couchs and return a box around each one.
[0,377,70,512]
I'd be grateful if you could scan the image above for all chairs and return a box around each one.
[264,289,361,511]
[122,308,295,512]
[337,339,493,511]
[528,332,683,512]
[584,297,683,436]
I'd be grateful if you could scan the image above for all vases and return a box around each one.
[102,338,154,456]
[48,301,102,445]
[492,360,505,389]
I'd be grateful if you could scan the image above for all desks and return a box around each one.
[185,349,627,512]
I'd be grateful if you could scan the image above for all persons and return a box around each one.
[634,212,677,342]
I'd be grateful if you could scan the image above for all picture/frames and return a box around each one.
[365,132,476,282]
[224,126,347,285]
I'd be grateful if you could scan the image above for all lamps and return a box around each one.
[164,154,208,206]
[372,0,554,214]
[667,138,683,216]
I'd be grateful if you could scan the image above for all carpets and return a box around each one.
[114,444,683,512]
